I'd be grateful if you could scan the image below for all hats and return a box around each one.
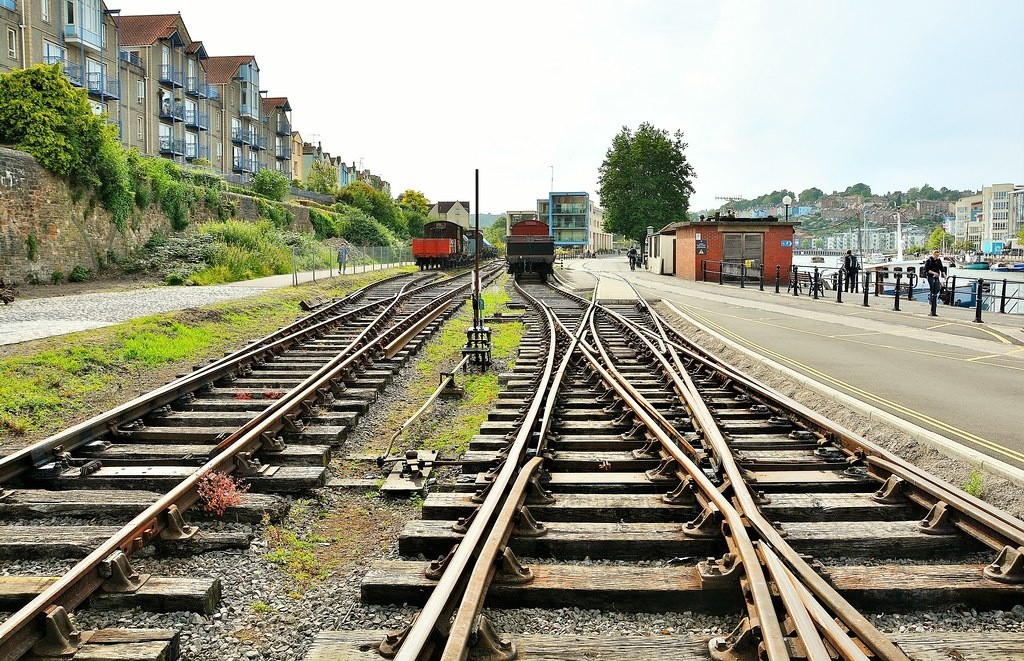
[847,250,851,254]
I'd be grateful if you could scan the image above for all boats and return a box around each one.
[1011,263,1024,272]
[990,262,1009,271]
[956,261,990,270]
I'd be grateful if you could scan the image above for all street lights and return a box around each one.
[782,195,793,221]
[549,166,554,191]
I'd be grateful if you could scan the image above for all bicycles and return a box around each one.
[628,255,636,272]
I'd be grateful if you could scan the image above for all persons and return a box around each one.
[923,250,947,307]
[842,249,860,293]
[627,246,637,270]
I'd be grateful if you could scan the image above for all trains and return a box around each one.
[412,218,498,269]
[505,219,557,282]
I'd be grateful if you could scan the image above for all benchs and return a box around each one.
[785,269,824,297]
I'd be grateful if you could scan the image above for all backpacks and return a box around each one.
[923,258,932,275]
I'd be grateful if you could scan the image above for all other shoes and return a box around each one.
[851,291,853,293]
[844,290,848,292]
